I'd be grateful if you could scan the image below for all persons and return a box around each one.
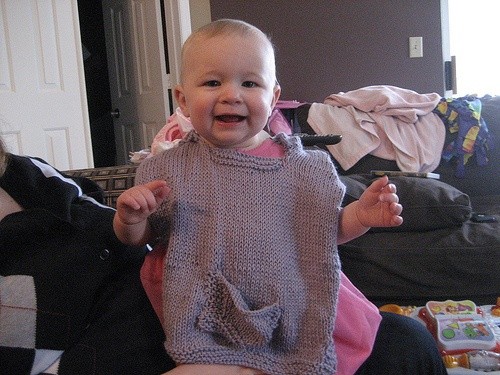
[110,19,403,375]
[0,135,175,373]
[150,87,453,375]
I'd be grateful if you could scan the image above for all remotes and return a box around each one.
[287,133,343,146]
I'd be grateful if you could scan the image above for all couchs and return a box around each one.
[297,96,500,307]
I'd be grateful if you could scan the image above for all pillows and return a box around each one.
[340,170,471,233]
